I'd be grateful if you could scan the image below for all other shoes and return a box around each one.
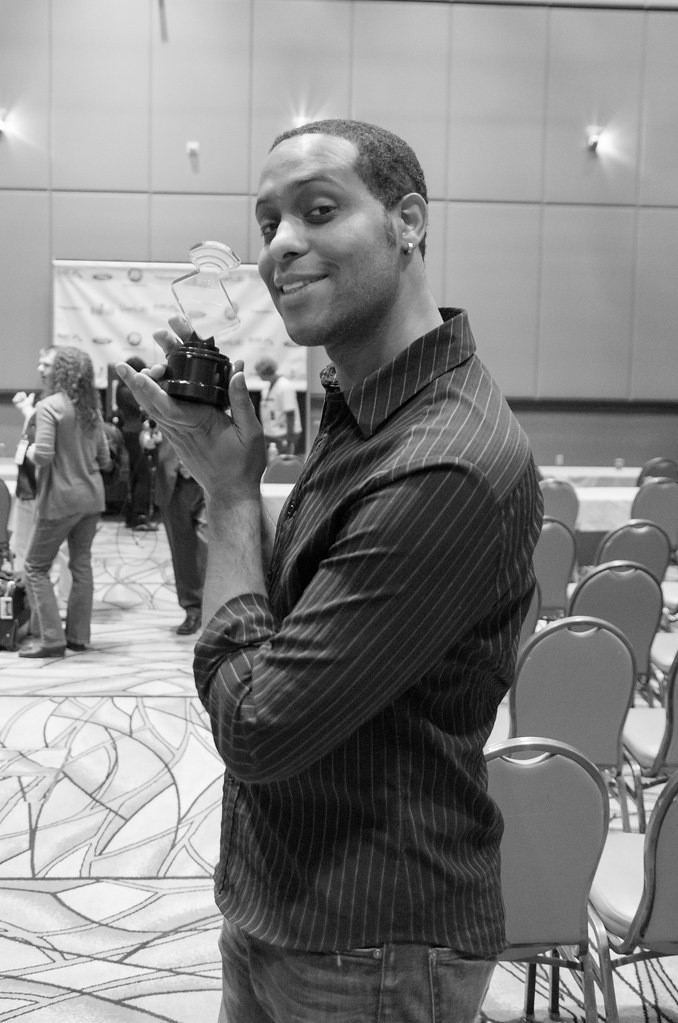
[19,645,65,657]
[132,521,159,532]
[66,640,83,651]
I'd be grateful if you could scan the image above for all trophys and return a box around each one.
[157,241,242,409]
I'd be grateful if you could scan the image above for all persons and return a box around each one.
[140,420,209,635]
[115,357,157,532]
[115,121,544,1023]
[253,356,302,459]
[12,345,110,657]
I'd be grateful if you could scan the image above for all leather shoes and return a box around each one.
[176,616,200,635]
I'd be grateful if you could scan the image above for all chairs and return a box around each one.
[477,458,677,1023]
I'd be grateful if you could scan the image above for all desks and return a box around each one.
[538,466,644,487]
[571,483,640,584]
